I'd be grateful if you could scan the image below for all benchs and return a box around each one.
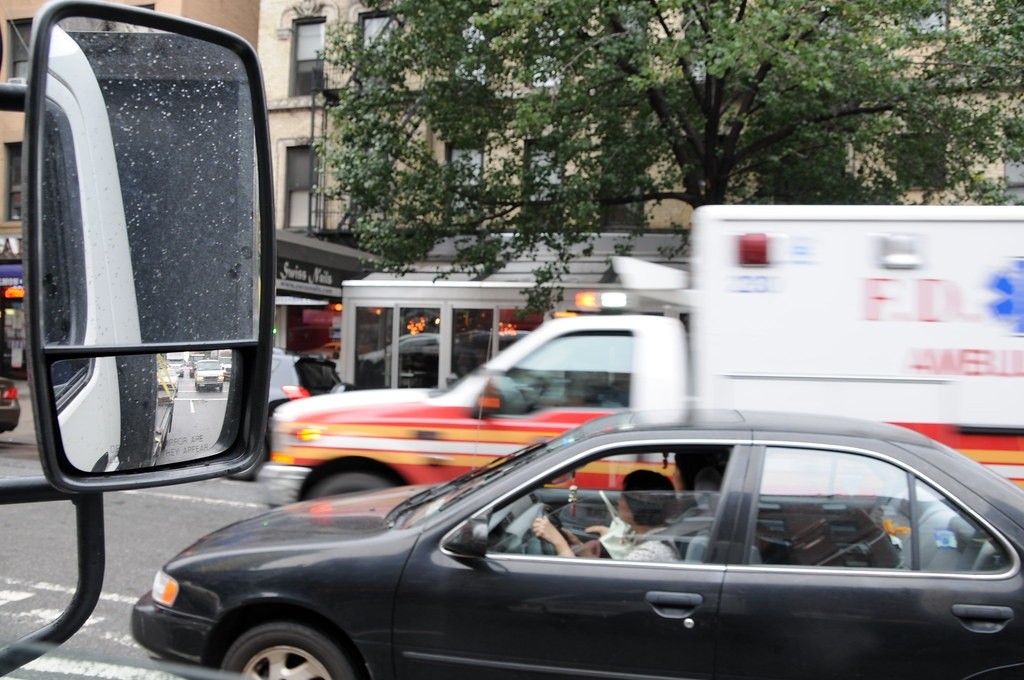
[882,485,1002,575]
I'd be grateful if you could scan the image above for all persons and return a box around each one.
[675,451,699,517]
[532,471,684,562]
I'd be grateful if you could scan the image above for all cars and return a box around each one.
[155,351,233,406]
[123,405,1024,680]
[220,343,303,482]
[357,328,532,390]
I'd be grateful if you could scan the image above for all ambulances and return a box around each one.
[254,197,1023,507]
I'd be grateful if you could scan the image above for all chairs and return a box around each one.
[684,466,721,563]
